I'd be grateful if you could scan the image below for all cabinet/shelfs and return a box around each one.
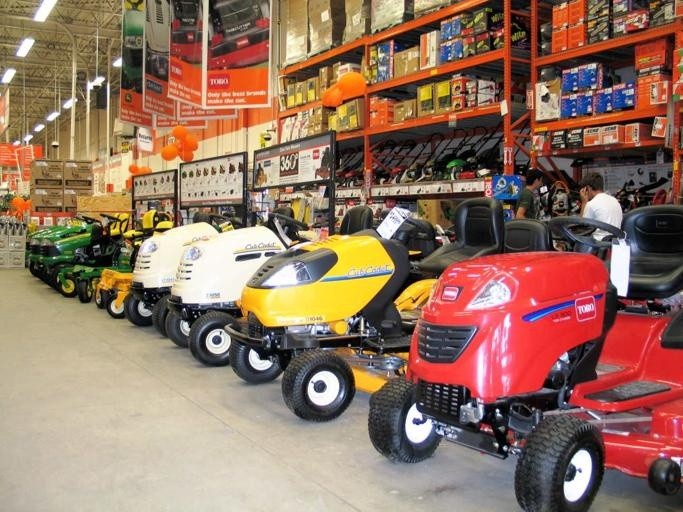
[266,0,683,210]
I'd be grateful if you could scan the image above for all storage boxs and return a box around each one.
[27,157,95,213]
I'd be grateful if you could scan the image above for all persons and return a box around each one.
[515,169,544,220]
[578,172,623,242]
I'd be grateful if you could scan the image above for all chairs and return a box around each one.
[191,212,212,226]
[339,204,374,235]
[399,199,548,275]
[109,209,160,240]
[263,207,294,233]
[613,203,682,296]
